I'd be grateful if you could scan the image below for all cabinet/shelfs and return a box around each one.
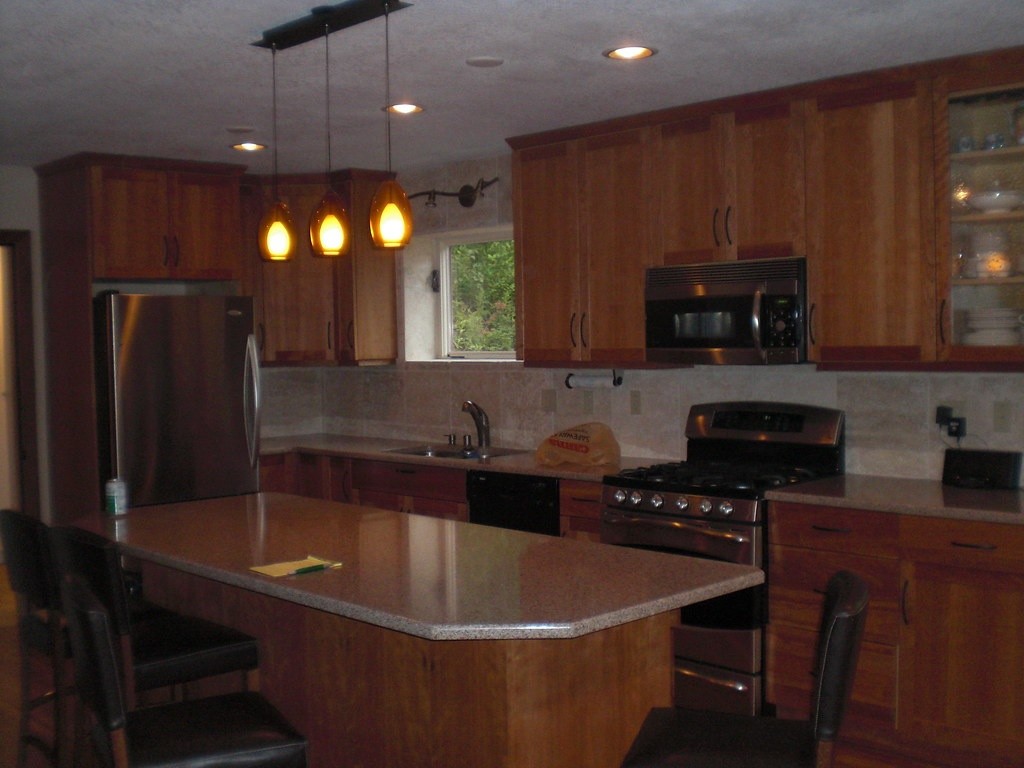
[34,152,249,283]
[259,451,468,522]
[768,500,1024,767]
[242,167,400,368]
[505,41,1024,375]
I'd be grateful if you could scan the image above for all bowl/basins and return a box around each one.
[968,190,1022,214]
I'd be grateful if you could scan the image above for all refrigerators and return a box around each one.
[92,291,263,511]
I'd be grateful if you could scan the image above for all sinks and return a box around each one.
[382,444,530,460]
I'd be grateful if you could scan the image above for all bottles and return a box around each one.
[951,181,971,213]
[1014,106,1024,146]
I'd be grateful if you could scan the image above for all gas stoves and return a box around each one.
[602,402,847,499]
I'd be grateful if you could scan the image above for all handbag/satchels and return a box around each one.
[536,421,618,471]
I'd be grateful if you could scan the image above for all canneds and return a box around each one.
[105,478,127,516]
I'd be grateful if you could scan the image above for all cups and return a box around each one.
[956,132,1005,153]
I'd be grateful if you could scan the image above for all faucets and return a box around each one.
[462,400,492,458]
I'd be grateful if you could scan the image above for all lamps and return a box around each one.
[409,175,500,209]
[259,43,298,263]
[367,2,414,250]
[307,22,352,259]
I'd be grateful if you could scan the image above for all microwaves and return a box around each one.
[644,256,809,367]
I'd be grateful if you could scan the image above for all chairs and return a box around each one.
[0,507,311,768]
[617,567,868,768]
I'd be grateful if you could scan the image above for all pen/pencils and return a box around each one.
[288,564,333,575]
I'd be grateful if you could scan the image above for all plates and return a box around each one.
[962,230,1017,278]
[959,308,1024,347]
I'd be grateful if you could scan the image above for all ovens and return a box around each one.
[601,484,768,718]
